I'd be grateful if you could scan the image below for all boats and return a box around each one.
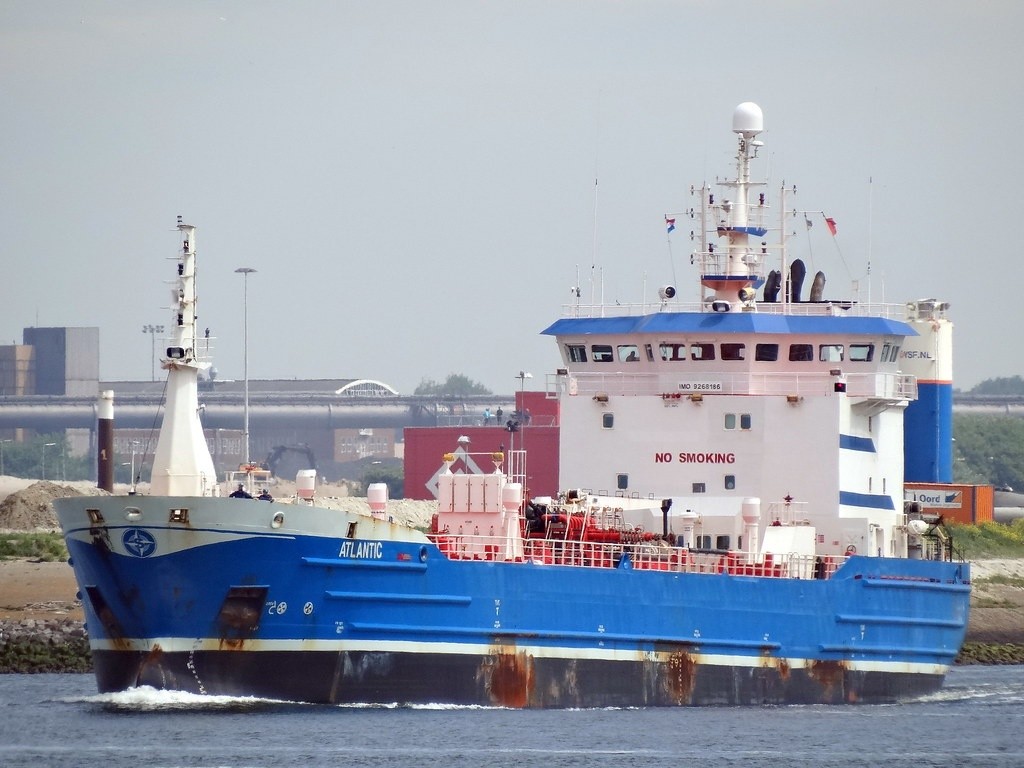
[49,101,971,717]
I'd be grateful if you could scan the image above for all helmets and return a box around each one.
[263,489,268,493]
[238,483,244,486]
[486,407,490,411]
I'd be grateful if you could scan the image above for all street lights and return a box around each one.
[233,268,260,470]
[513,369,532,453]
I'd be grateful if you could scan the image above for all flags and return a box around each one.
[666,219,675,234]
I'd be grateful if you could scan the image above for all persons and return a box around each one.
[497,407,503,425]
[229,483,253,499]
[483,408,490,426]
[815,557,827,580]
[259,489,272,501]
[626,351,635,362]
